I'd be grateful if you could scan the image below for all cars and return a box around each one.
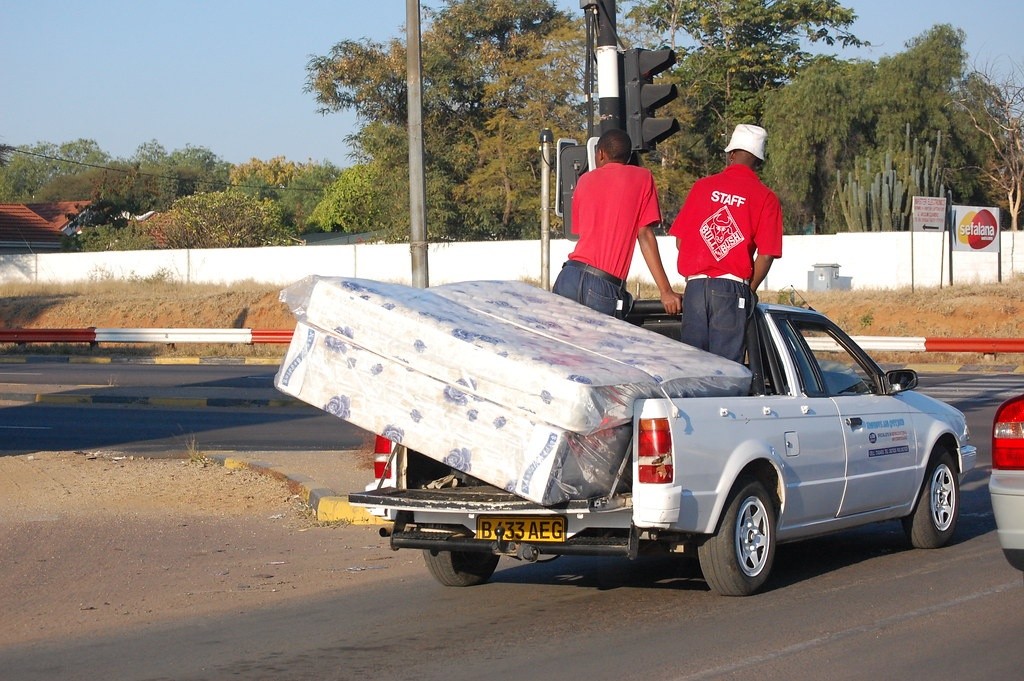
[987,393,1024,571]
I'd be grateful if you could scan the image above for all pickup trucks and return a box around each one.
[344,290,980,597]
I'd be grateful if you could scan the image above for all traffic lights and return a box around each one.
[620,46,681,155]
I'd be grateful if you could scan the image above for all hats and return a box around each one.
[723,124,767,162]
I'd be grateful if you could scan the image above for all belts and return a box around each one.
[686,272,750,287]
[562,259,627,290]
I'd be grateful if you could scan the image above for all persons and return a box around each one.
[553,128,681,322]
[668,124,782,364]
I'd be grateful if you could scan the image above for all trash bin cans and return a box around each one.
[812,263,841,292]
